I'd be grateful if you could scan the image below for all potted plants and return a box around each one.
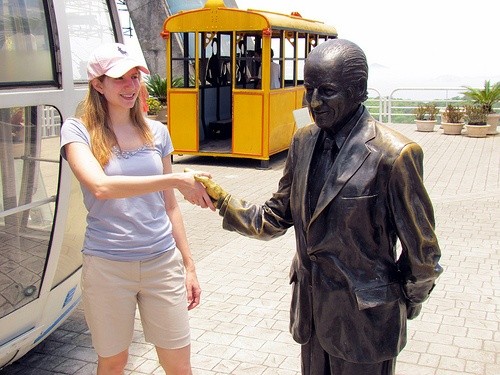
[143,73,198,124]
[413,80,500,137]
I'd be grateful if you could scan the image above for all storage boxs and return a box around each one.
[209,118,232,140]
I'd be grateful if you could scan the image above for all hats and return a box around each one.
[87,43,150,84]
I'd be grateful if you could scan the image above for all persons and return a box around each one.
[257,49,281,90]
[59,43,213,375]
[184,40,444,375]
